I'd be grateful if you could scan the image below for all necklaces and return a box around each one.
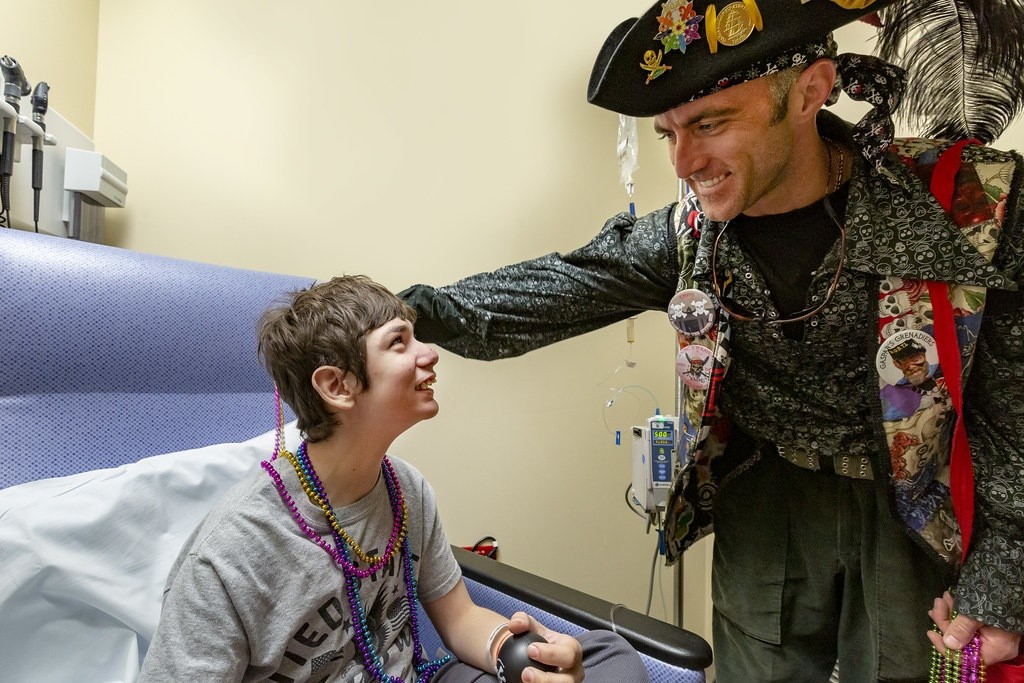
[263,379,450,683]
[688,200,846,324]
[819,131,845,194]
[929,606,987,683]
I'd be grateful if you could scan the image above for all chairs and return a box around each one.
[0,225,714,683]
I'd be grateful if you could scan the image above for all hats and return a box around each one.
[586,0,900,119]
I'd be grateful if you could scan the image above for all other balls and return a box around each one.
[497,631,559,683]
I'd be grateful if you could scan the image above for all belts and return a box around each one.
[725,422,873,481]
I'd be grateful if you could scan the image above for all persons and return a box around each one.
[134,276,582,683]
[394,0,1024,683]
